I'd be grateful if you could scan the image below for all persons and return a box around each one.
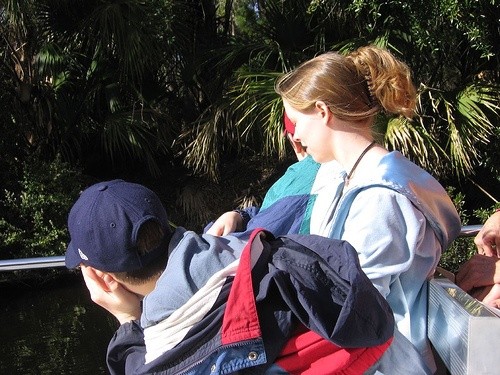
[205,110,345,238]
[455,210,500,310]
[275,45,461,375]
[67,180,433,375]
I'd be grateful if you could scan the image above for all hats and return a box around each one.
[283,109,295,135]
[64,179,173,271]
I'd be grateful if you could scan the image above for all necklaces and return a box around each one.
[345,140,379,186]
